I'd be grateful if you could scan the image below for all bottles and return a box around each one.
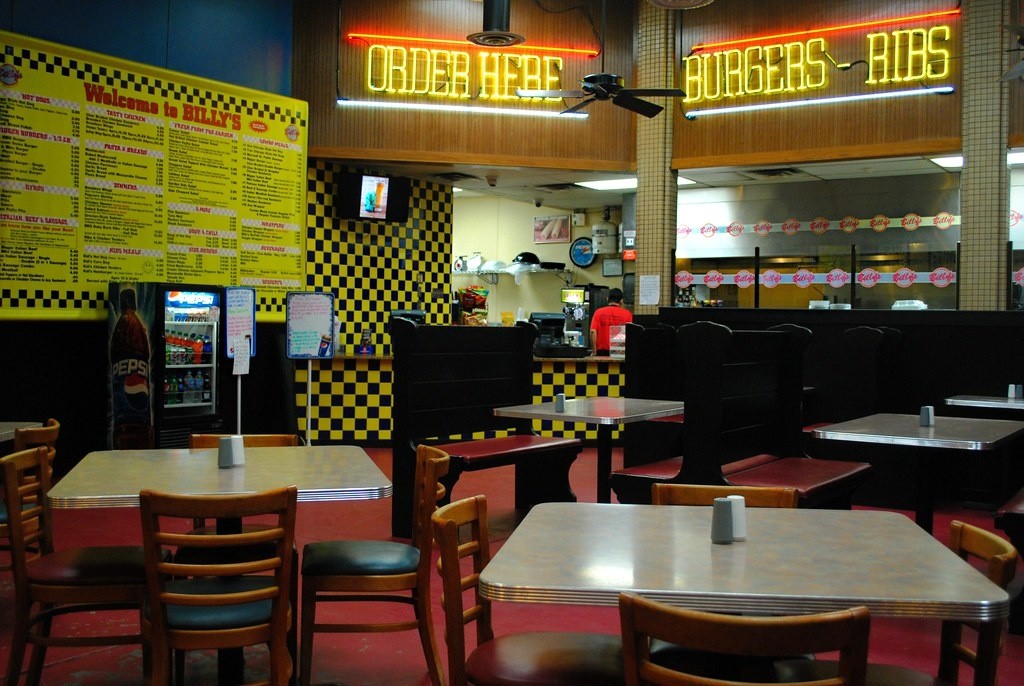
[163,328,213,405]
[920,407,930,426]
[727,494,746,542]
[1008,384,1016,399]
[230,435,245,466]
[110,288,155,450]
[218,438,231,468]
[926,406,935,426]
[712,497,733,545]
[556,394,564,412]
[1015,384,1023,398]
[676,287,697,307]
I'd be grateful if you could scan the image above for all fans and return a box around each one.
[516,0,688,118]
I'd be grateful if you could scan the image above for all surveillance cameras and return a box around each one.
[487,179,496,186]
[534,200,543,207]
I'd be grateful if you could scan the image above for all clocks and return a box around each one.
[569,236,598,268]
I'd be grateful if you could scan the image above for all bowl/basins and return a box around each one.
[809,300,830,310]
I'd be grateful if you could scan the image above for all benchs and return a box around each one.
[390,315,583,544]
[609,320,872,509]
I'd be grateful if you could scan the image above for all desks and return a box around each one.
[477,506,1013,624]
[944,395,1024,411]
[812,413,1024,537]
[493,397,685,503]
[46,446,394,686]
[0,421,44,443]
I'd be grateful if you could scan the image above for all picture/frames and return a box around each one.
[533,214,572,244]
[602,258,624,277]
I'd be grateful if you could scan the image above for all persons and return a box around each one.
[590,288,633,356]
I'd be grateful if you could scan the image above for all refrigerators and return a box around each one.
[108,280,222,450]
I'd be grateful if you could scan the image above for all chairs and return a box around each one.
[139,485,298,686]
[617,590,870,686]
[175,435,299,680]
[300,445,450,686]
[0,447,174,686]
[14,418,60,491]
[650,482,798,509]
[430,493,628,686]
[775,519,1017,686]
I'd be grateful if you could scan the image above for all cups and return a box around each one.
[317,338,332,357]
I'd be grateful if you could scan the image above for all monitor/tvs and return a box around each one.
[336,172,411,222]
